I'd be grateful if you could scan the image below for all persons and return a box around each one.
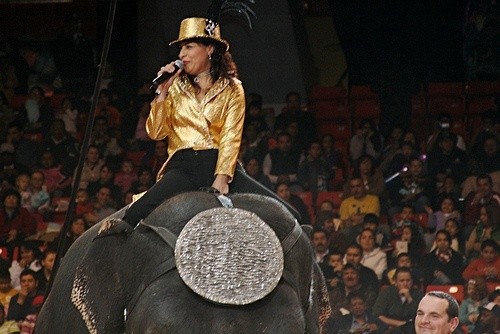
[0,35,500,334]
[121,0,303,230]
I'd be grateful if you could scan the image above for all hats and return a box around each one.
[169,17,229,51]
[32,296,45,305]
[479,302,500,316]
[343,262,359,271]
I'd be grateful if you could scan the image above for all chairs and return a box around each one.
[0,85,500,303]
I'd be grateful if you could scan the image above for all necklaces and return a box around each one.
[194,71,213,83]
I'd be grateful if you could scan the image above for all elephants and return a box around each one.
[34,191,322,334]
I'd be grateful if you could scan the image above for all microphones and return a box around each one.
[150,60,184,89]
